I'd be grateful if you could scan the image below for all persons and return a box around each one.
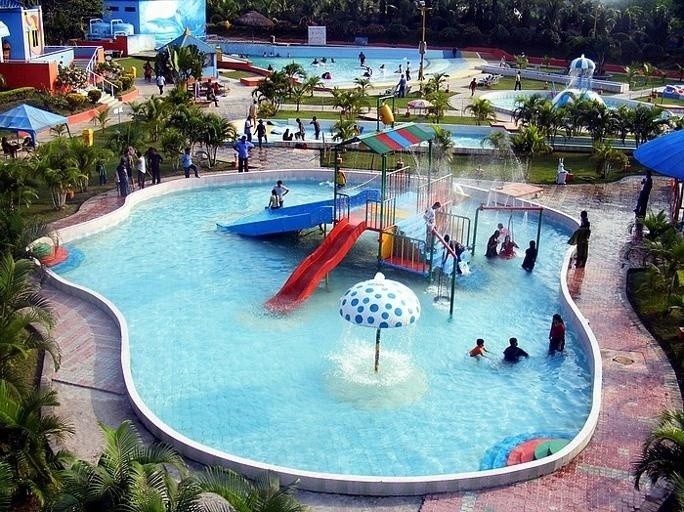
[498,55,506,67]
[232,99,269,173]
[633,170,653,216]
[143,61,166,95]
[267,64,273,70]
[310,56,335,79]
[295,118,306,141]
[514,70,521,91]
[282,128,293,142]
[502,337,529,362]
[467,338,491,361]
[548,313,567,357]
[574,210,590,269]
[181,146,201,178]
[309,115,321,140]
[484,222,538,274]
[113,146,163,198]
[423,201,465,278]
[469,78,478,97]
[264,178,289,210]
[240,52,250,59]
[262,51,281,58]
[336,165,347,188]
[196,78,224,107]
[2,136,34,159]
[358,51,426,98]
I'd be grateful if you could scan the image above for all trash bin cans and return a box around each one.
[81,129,93,147]
[129,66,136,77]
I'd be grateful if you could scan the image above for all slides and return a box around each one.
[263,218,366,315]
[215,189,381,237]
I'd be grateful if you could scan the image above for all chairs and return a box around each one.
[379,86,397,96]
[1,145,17,161]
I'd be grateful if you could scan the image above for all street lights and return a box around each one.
[414,0,434,82]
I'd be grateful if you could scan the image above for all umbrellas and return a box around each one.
[570,57,596,89]
[338,278,421,372]
[632,129,684,222]
[232,11,274,45]
[406,99,435,115]
